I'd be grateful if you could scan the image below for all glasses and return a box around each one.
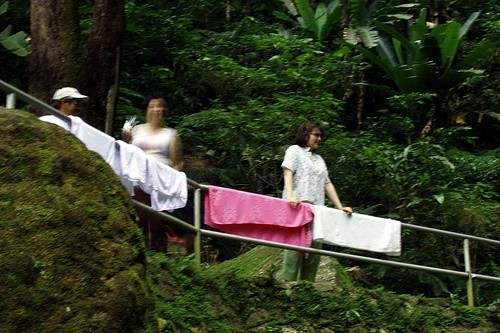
[312,132,324,138]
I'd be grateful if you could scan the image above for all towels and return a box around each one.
[205,180,312,247]
[65,116,116,166]
[119,137,189,213]
[309,202,405,260]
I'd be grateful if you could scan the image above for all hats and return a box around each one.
[53,87,89,104]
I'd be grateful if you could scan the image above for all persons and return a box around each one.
[39,87,89,132]
[121,94,185,253]
[281,122,352,282]
